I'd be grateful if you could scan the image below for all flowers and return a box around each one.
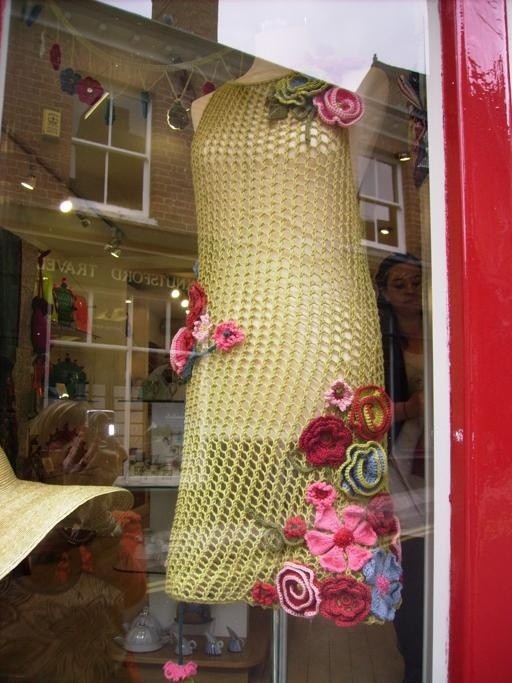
[262,71,369,151]
[246,376,407,628]
[166,279,246,381]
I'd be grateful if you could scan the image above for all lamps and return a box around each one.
[0,126,130,260]
[398,151,411,162]
[378,224,393,235]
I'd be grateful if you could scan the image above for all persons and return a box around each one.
[377,253,430,678]
[165,56,404,625]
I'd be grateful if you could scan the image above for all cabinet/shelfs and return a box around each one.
[113,468,270,683]
